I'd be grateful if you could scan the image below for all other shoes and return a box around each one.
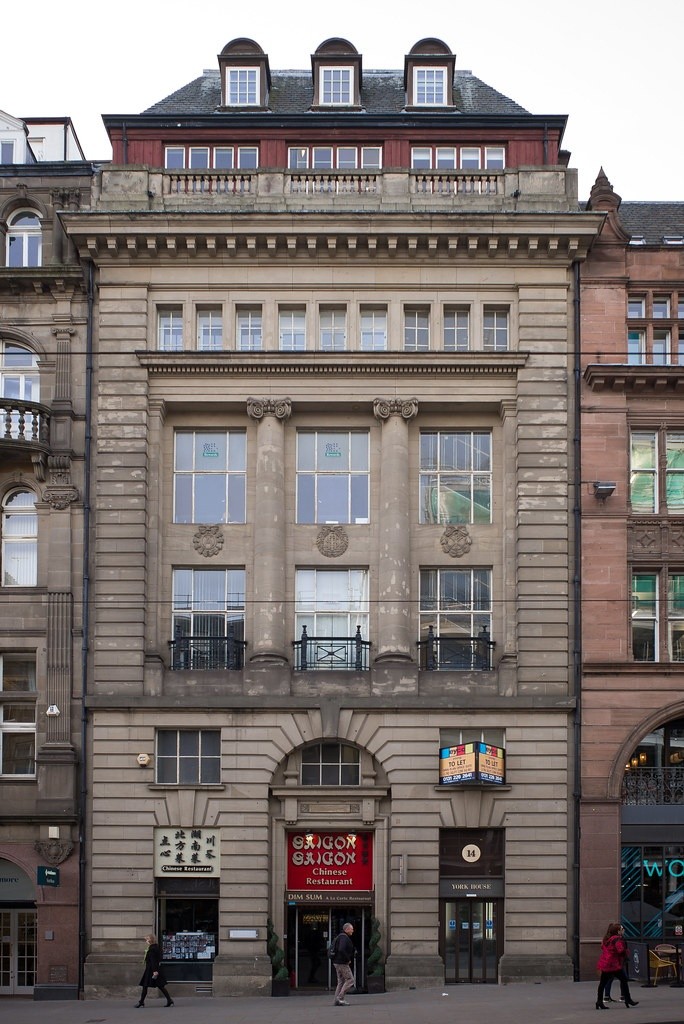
[338,999,350,1005]
[619,996,625,1002]
[603,998,617,1002]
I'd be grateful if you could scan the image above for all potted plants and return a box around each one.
[367,915,384,993]
[268,918,290,996]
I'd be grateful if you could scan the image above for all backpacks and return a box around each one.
[327,933,346,959]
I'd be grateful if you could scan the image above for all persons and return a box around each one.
[333,923,355,1006]
[134,933,174,1009]
[596,923,639,1010]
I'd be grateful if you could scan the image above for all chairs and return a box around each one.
[649,950,678,985]
[655,944,678,982]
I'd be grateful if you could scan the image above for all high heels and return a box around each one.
[134,1000,144,1008]
[625,999,639,1008]
[596,1002,610,1010]
[164,999,174,1007]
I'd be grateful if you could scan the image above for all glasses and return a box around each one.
[620,928,624,930]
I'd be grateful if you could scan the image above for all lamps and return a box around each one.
[228,929,259,940]
[593,482,616,499]
[624,762,631,771]
[632,752,639,767]
[639,747,647,766]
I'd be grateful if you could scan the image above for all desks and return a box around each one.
[656,949,682,984]
